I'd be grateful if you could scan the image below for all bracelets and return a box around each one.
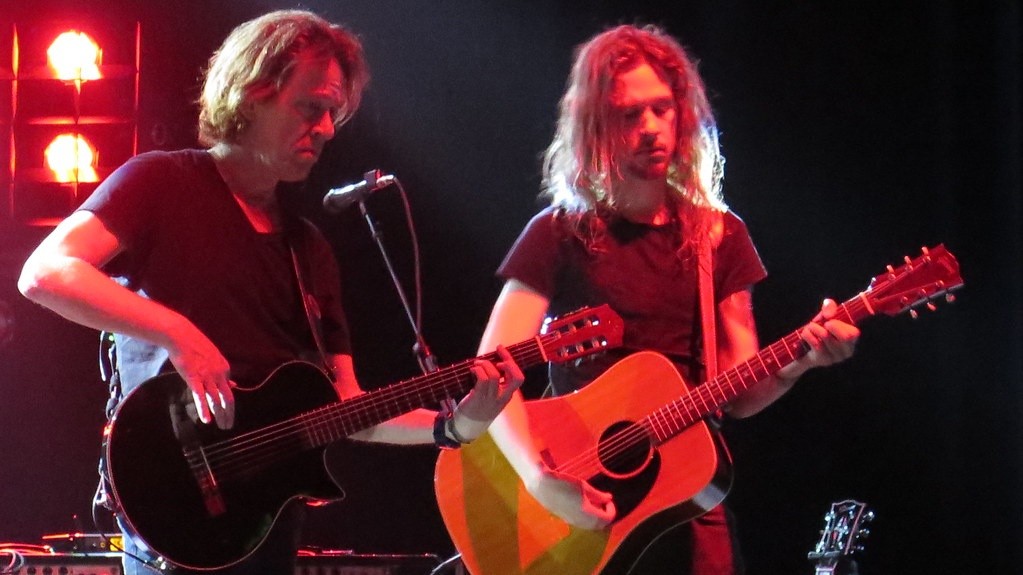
[447,411,473,444]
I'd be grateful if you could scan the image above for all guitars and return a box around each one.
[431,238,967,575]
[98,298,627,574]
[807,497,878,575]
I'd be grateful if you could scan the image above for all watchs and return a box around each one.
[433,412,461,450]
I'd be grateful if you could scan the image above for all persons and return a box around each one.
[18,11,526,575]
[473,25,860,575]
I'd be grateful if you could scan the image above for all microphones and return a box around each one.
[322,175,395,216]
